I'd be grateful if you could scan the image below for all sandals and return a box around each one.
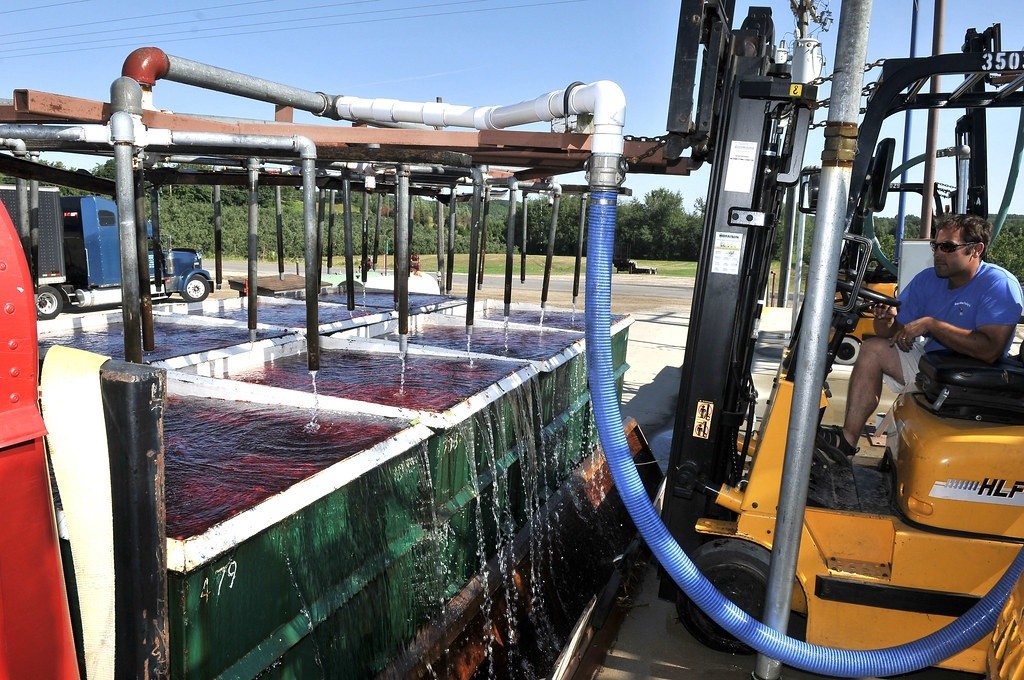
[817,426,859,467]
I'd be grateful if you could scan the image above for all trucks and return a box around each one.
[1,185,215,323]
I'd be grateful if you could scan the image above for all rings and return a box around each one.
[901,337,906,340]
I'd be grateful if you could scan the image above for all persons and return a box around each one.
[411,254,421,276]
[367,255,373,270]
[815,215,1024,466]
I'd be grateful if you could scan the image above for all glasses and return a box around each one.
[931,241,975,253]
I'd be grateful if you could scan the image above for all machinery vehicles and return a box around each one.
[655,1,1024,679]
